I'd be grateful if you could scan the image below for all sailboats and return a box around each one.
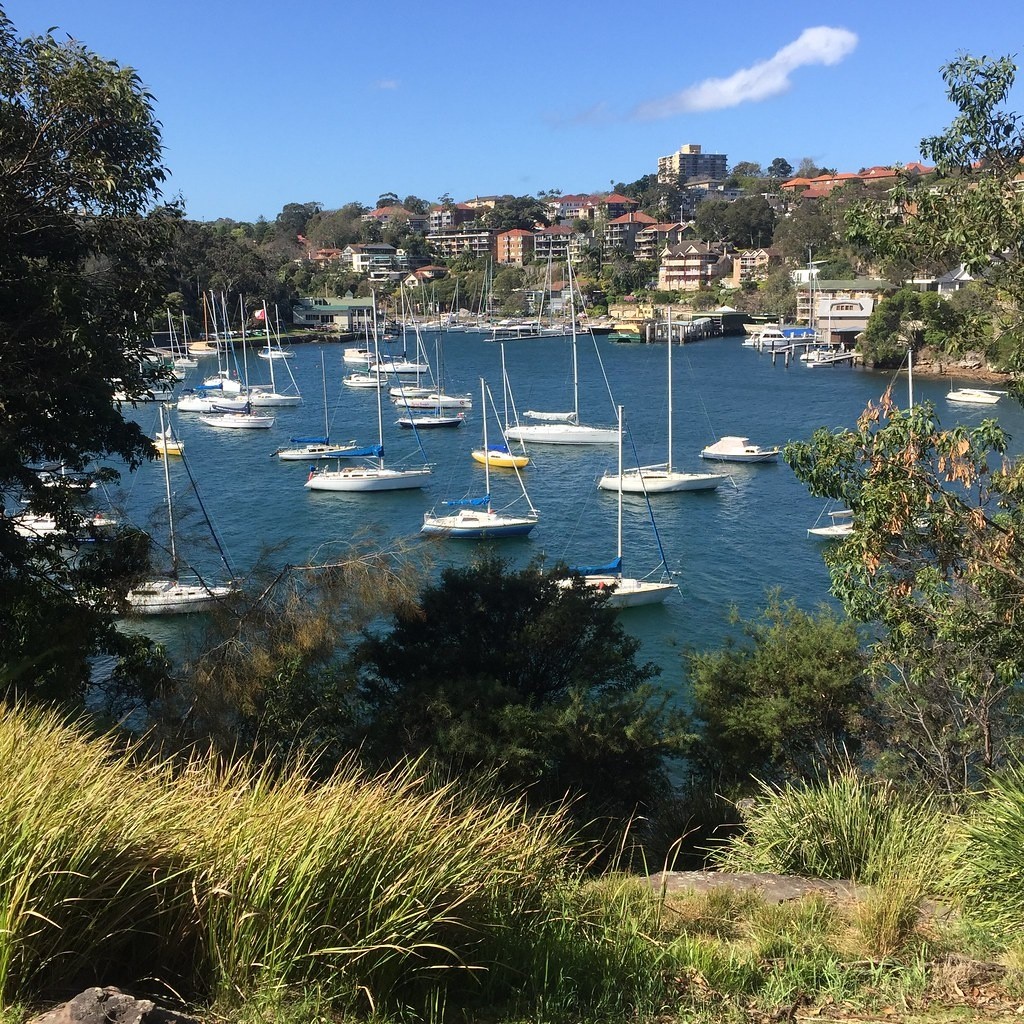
[71,402,246,614]
[109,286,304,431]
[597,307,731,495]
[304,243,564,490]
[419,377,541,538]
[268,349,379,462]
[500,244,628,446]
[522,404,681,608]
[808,346,940,539]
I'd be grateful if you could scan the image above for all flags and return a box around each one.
[254,308,265,320]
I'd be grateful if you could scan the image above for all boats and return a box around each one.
[698,434,781,463]
[943,388,1008,406]
[6,510,119,542]
[148,422,186,456]
[741,322,814,348]
[37,458,98,497]
